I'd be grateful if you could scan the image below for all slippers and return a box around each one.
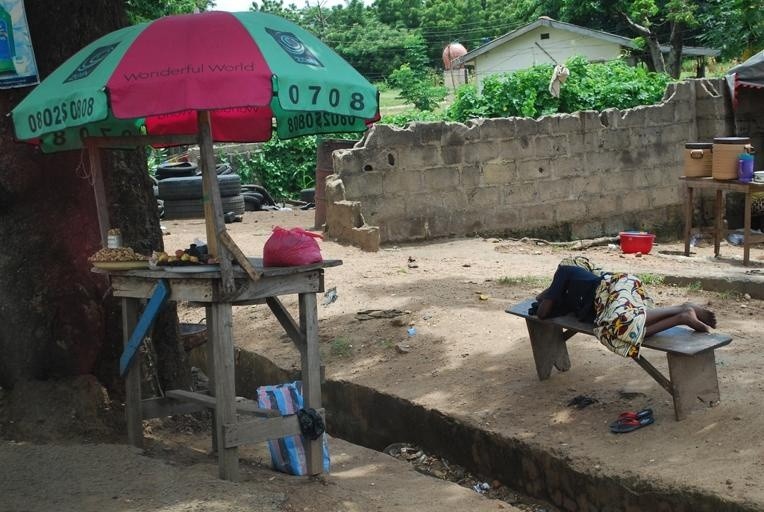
[609,409,654,433]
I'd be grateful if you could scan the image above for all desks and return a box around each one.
[677,175,764,266]
[89,255,343,484]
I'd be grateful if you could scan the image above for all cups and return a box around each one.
[754,170,764,183]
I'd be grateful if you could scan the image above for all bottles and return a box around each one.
[180,243,200,264]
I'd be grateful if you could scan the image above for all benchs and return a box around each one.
[504,295,733,422]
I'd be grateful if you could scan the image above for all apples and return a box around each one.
[159,249,198,263]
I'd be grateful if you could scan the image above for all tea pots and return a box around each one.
[735,153,753,183]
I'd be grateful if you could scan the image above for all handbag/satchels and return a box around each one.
[256,381,330,475]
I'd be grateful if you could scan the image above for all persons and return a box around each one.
[530,258,720,359]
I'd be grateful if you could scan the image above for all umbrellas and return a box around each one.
[4,9,381,261]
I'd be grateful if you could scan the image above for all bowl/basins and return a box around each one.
[620,234,656,254]
